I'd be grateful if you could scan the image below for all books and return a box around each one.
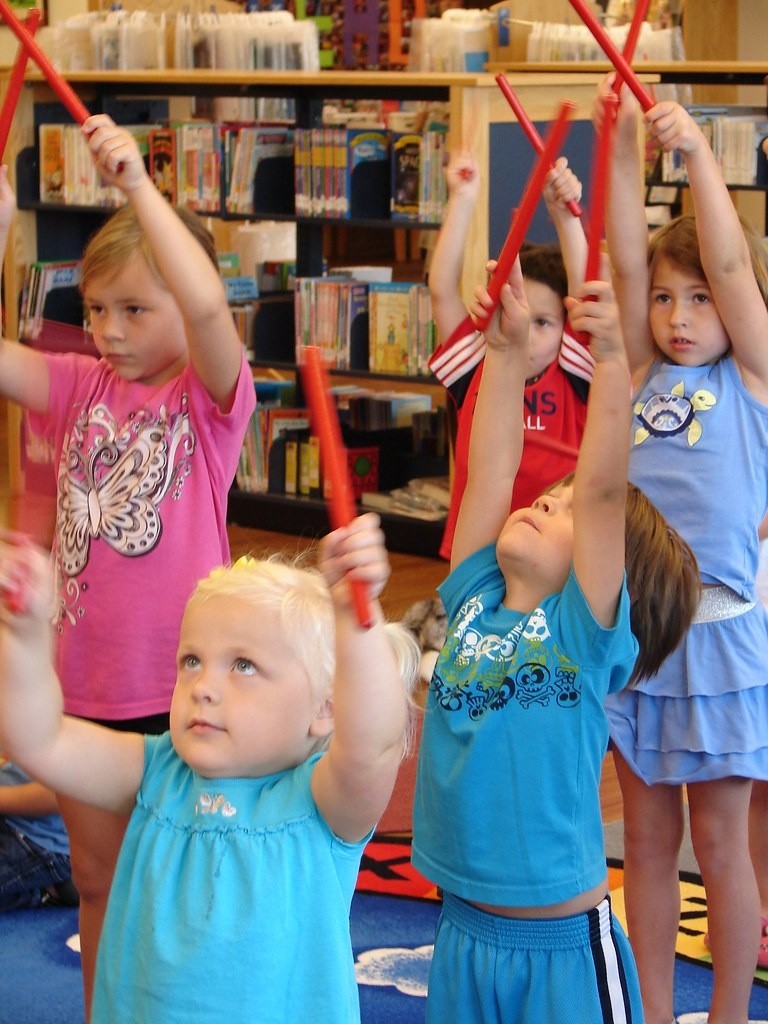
[654,111,768,190]
[27,92,459,528]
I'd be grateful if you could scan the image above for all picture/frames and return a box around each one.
[0,0,48,26]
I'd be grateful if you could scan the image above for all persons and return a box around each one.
[409,242,705,1024]
[426,150,615,577]
[569,69,768,1024]
[4,113,259,1024]
[0,507,416,1024]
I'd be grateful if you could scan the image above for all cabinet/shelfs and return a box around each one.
[19,59,662,569]
[483,59,768,251]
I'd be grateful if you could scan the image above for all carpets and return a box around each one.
[1,831,767,1023]
